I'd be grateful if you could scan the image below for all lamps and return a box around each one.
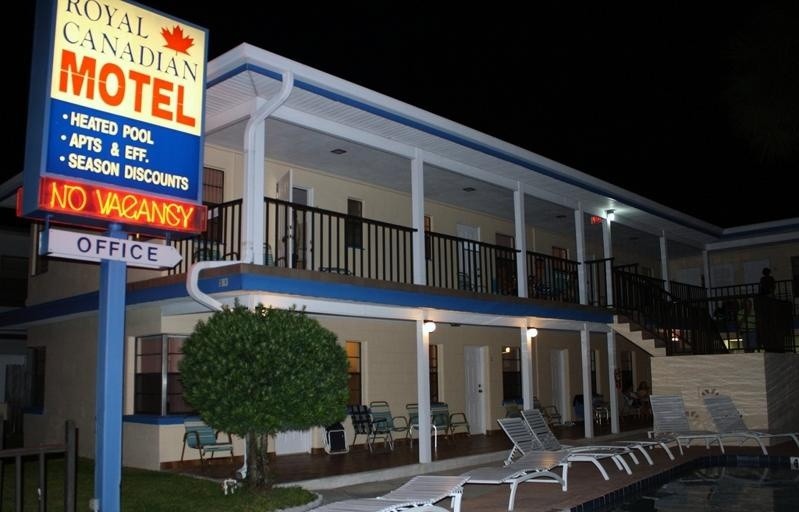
[424,319,436,334]
[603,208,617,222]
[527,327,538,338]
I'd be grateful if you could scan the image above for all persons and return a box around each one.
[759,267,776,298]
[637,381,651,420]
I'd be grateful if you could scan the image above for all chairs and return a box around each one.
[700,391,799,455]
[646,392,725,453]
[344,398,473,451]
[448,448,571,511]
[192,239,355,277]
[454,269,564,299]
[494,390,677,481]
[180,415,235,467]
[301,472,473,512]
